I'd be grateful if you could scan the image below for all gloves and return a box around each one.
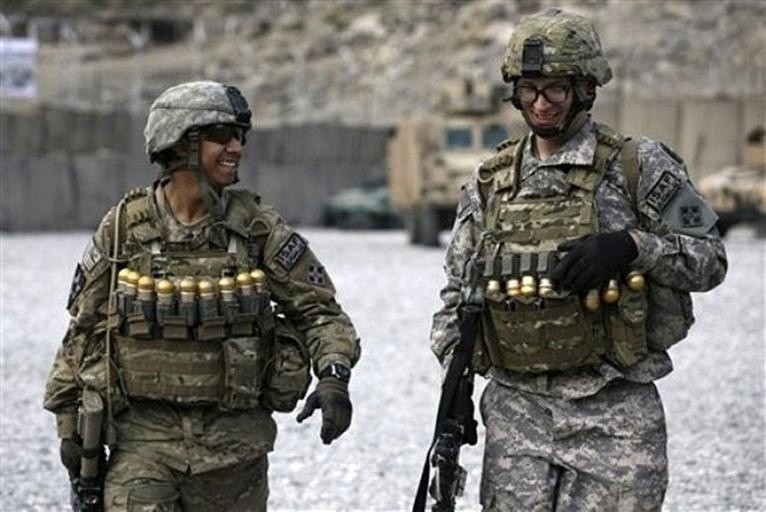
[553,231,640,293]
[296,378,353,445]
[449,381,477,446]
[62,439,109,479]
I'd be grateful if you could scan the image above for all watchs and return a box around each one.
[316,364,352,385]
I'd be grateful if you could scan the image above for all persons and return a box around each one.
[39,80,364,512]
[428,7,729,511]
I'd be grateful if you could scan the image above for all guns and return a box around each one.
[429,305,483,511]
[76,385,103,512]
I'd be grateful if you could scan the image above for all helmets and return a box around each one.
[500,9,612,87]
[144,80,251,161]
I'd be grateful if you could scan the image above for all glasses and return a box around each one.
[515,81,572,104]
[203,124,248,144]
